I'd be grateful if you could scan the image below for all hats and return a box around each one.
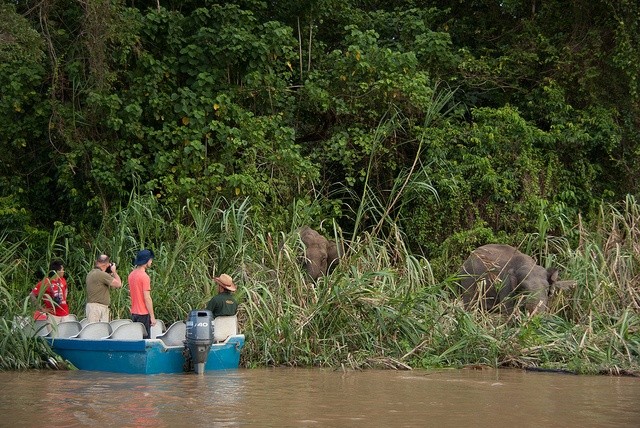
[215,274,237,291]
[134,249,155,265]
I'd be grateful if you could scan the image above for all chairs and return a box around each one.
[80,318,89,326]
[103,322,148,340]
[69,314,78,321]
[150,319,166,339]
[20,320,52,337]
[70,321,113,338]
[213,315,239,343]
[110,318,133,330]
[42,322,82,337]
[140,322,148,338]
[156,321,187,346]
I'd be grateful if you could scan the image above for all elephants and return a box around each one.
[457,243,577,317]
[278,225,343,286]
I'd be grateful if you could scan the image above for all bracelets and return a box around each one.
[39,307,42,311]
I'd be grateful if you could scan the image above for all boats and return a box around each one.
[7,312,245,375]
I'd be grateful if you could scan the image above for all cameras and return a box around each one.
[106,263,115,274]
[52,296,61,308]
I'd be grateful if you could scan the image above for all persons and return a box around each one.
[30,261,69,325]
[206,274,237,319]
[85,254,121,322]
[128,249,156,339]
